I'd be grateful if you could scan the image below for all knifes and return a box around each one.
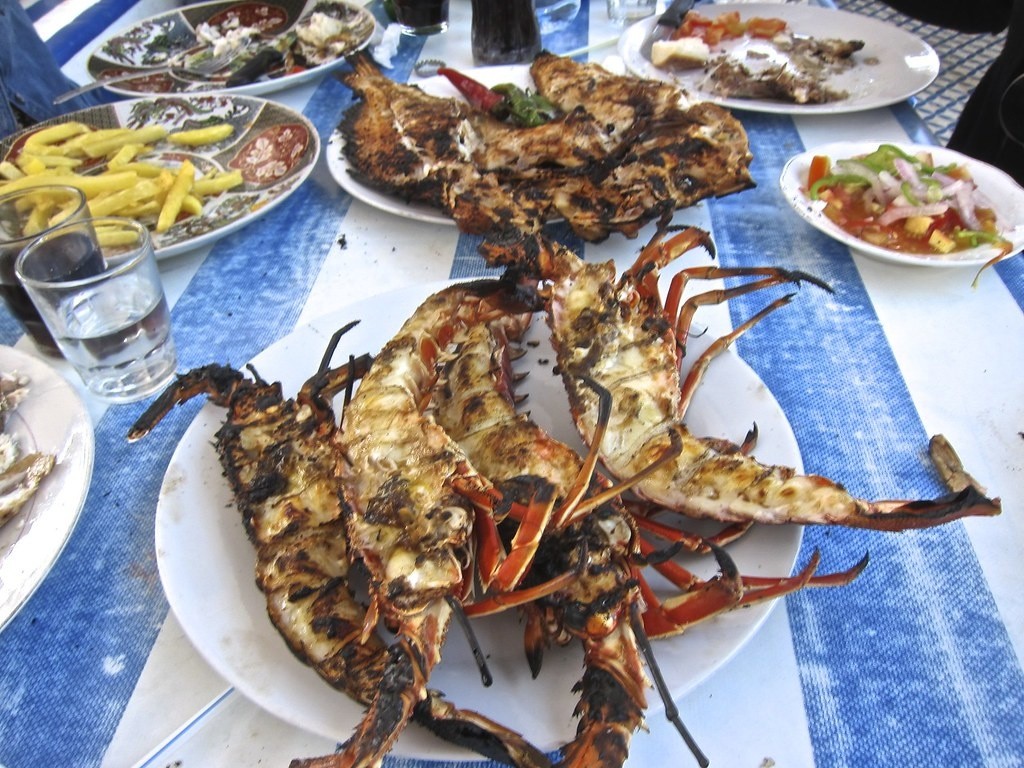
[642,0,696,61]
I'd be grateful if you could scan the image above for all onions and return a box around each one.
[832,157,992,232]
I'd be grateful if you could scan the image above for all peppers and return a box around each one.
[954,230,1000,245]
[437,68,525,121]
[808,145,959,207]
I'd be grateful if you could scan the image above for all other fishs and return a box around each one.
[333,46,758,246]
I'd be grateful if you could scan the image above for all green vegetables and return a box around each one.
[491,83,559,125]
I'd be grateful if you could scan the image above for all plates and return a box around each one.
[87,0,375,98]
[1,93,321,266]
[328,65,567,223]
[619,2,939,114]
[156,277,808,763]
[0,345,95,632]
[780,141,1024,267]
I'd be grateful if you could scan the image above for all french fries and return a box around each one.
[0,121,244,245]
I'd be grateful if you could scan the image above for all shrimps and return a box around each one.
[125,223,1005,768]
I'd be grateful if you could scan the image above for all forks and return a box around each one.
[54,35,250,106]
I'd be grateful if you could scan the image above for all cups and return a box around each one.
[470,0,542,67]
[0,184,106,358]
[607,0,657,25]
[15,219,180,405]
[392,0,449,36]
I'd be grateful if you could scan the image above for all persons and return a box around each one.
[874,0,1024,190]
[0,0,109,142]
